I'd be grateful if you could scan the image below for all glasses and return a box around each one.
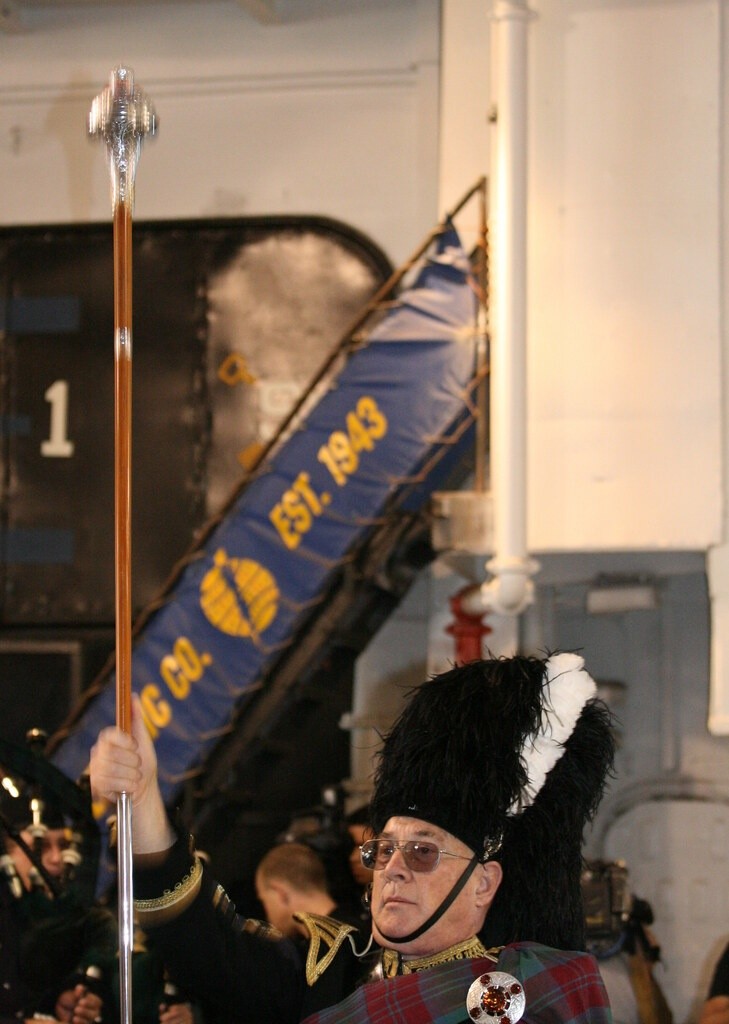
[359,839,475,874]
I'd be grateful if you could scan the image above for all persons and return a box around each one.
[0,758,641,1024]
[87,651,613,1024]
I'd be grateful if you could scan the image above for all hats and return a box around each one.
[367,647,616,950]
